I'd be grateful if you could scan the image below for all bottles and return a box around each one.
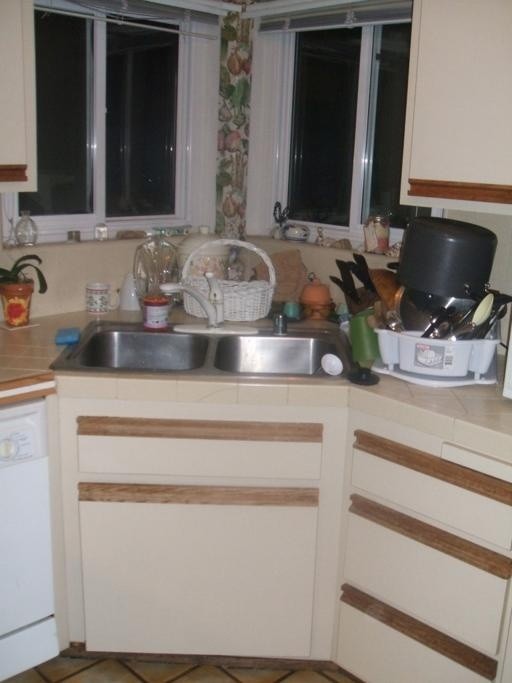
[364,214,391,254]
[16,210,38,246]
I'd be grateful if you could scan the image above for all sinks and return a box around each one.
[48,320,211,373]
[211,328,358,375]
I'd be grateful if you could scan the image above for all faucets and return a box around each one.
[159,282,218,327]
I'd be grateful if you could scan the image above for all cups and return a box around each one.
[122,272,145,311]
[86,282,121,316]
[143,293,171,332]
[351,312,381,362]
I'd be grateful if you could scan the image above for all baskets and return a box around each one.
[180,238,276,322]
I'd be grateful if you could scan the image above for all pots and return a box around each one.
[178,225,231,279]
[386,216,511,304]
[393,284,508,339]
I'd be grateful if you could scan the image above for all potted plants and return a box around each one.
[0,254,48,326]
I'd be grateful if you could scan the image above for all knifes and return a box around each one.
[328,253,374,304]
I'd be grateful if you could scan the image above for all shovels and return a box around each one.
[328,253,399,311]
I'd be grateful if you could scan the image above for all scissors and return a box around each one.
[273,202,291,227]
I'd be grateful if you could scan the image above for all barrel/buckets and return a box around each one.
[0,279,34,327]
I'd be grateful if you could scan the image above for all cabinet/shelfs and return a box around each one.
[0,0,38,192]
[399,0,512,215]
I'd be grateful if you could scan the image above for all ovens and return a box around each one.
[1,398,63,642]
